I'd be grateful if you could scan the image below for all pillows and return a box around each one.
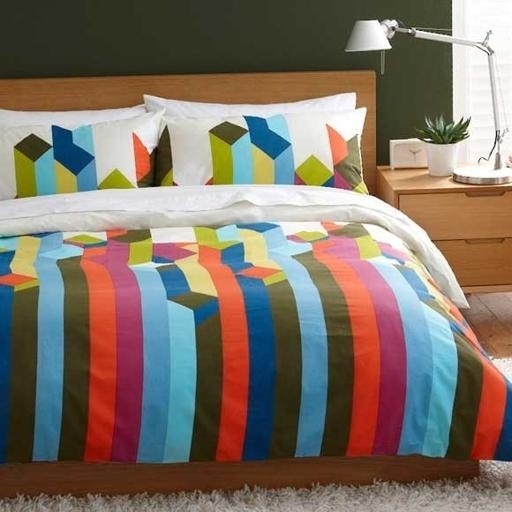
[1,103,147,126]
[1,109,173,199]
[143,92,356,115]
[161,106,367,193]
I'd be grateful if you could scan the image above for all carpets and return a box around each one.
[1,358,512,512]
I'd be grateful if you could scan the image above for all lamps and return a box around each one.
[343,17,512,184]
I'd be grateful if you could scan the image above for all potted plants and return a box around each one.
[414,114,471,178]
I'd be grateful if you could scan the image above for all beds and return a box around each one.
[0,68,512,496]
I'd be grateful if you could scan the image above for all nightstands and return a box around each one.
[376,164,512,293]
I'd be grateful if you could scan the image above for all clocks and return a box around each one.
[389,139,429,170]
